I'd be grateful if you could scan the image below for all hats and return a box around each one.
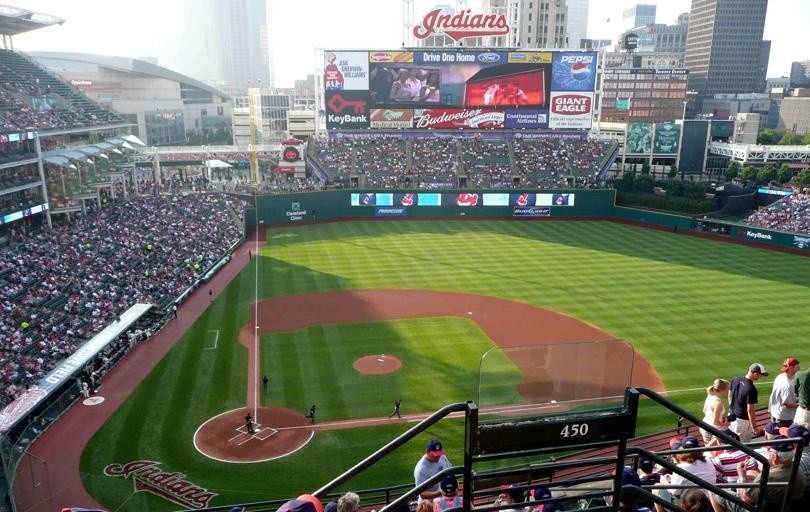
[762,422,810,452]
[781,358,799,372]
[276,494,323,512]
[530,488,551,500]
[719,428,734,439]
[682,436,698,449]
[613,467,640,486]
[440,475,458,493]
[499,483,524,498]
[670,438,682,450]
[749,363,768,376]
[427,440,443,457]
